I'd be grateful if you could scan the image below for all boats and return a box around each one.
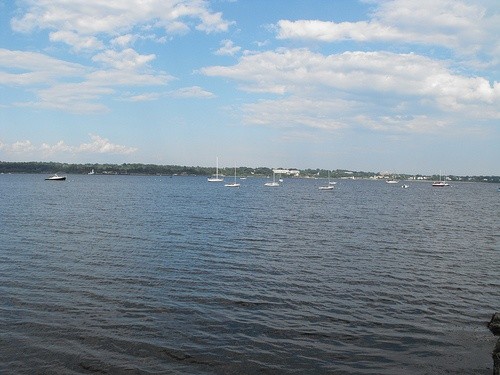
[240,177,247,180]
[385,179,399,184]
[431,180,450,187]
[44,173,66,180]
[329,181,338,186]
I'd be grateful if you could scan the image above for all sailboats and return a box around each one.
[264,170,280,187]
[319,171,338,191]
[223,167,243,188]
[207,155,227,182]
[278,168,284,183]
